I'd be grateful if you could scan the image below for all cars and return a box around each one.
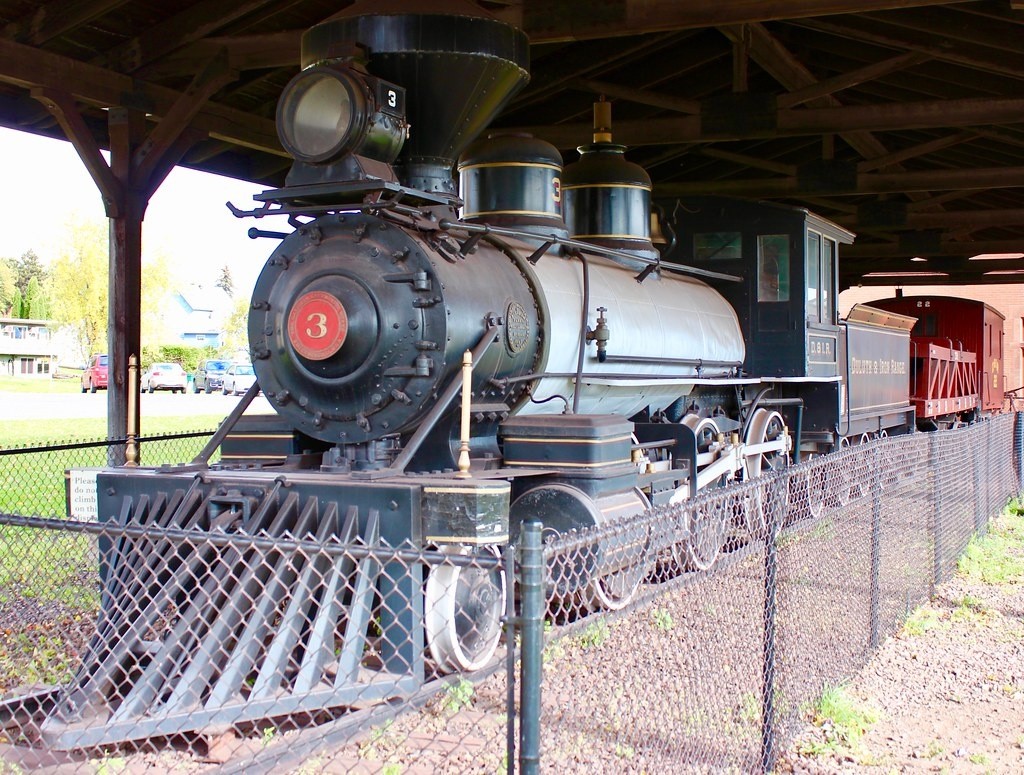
[193,360,230,394]
[80,353,108,394]
[222,365,261,397]
[140,363,188,395]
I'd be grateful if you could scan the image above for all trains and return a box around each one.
[92,5,1007,674]
